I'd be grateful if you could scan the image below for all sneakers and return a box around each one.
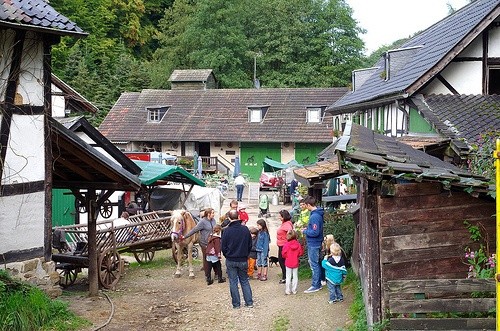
[304,285,323,293]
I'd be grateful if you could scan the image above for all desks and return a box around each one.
[258,185,281,205]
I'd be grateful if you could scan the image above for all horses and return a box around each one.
[168,208,202,281]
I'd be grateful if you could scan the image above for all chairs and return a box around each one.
[282,187,291,205]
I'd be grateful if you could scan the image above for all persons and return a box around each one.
[234,173,247,202]
[222,210,253,308]
[336,178,348,195]
[113,212,141,243]
[179,200,270,285]
[290,178,298,209]
[301,196,325,293]
[322,243,347,304]
[282,230,304,295]
[126,200,138,216]
[320,234,348,286]
[294,199,311,237]
[276,210,293,283]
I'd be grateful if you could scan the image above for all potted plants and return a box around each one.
[190,160,208,170]
[180,159,191,172]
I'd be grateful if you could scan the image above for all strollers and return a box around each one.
[258,194,271,218]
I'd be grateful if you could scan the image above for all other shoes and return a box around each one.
[255,274,261,279]
[339,299,343,302]
[261,275,268,281]
[214,274,218,280]
[279,279,286,283]
[208,278,213,285]
[220,277,226,283]
[329,300,336,304]
[247,305,253,308]
[321,280,327,285]
[234,306,240,309]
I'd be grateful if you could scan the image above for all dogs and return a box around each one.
[268,255,280,267]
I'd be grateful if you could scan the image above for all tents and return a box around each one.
[263,158,301,185]
